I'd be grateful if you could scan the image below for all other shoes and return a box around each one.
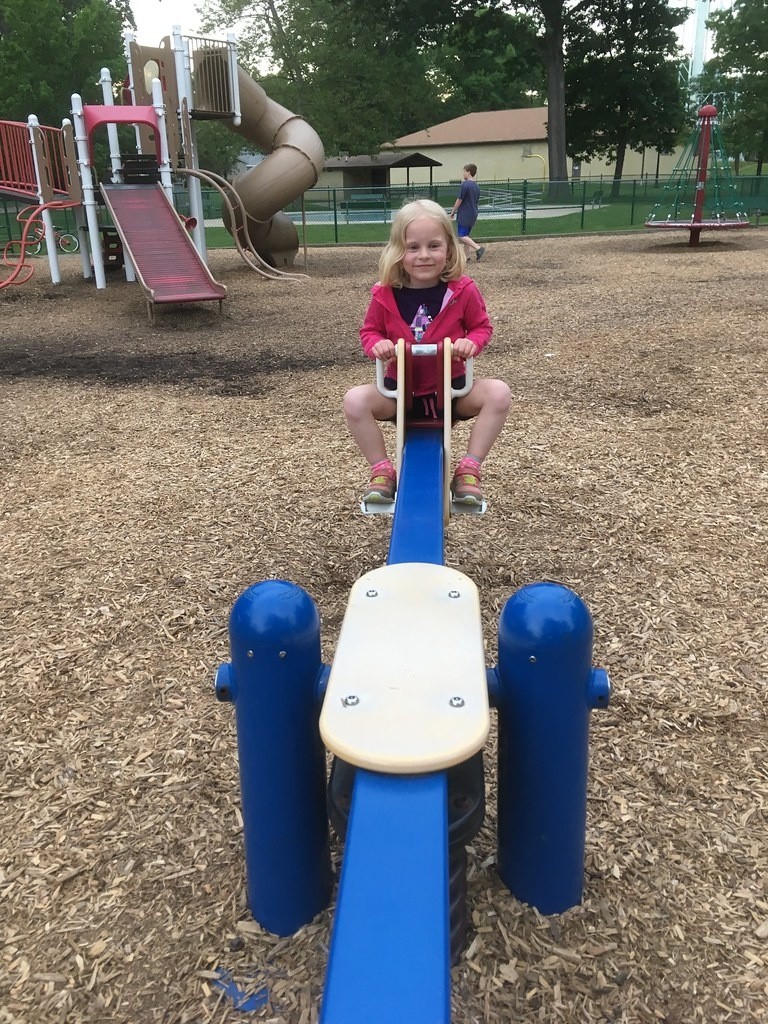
[466,257,470,262]
[476,247,485,260]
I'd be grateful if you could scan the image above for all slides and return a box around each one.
[193,48,326,266]
[99,181,227,304]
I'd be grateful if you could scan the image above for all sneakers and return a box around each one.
[363,469,396,503]
[450,466,482,505]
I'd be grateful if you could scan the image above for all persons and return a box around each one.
[451,164,485,261]
[342,200,511,506]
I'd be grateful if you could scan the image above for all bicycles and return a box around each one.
[19,217,79,255]
